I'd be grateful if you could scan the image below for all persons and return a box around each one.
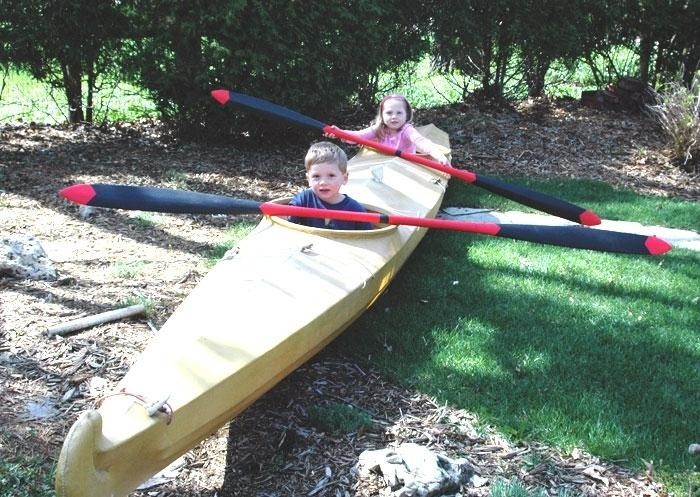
[279,140,373,231]
[320,92,453,181]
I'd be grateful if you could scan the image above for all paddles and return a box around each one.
[57,183,673,256]
[210,90,602,226]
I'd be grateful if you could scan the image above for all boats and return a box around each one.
[51,124,455,495]
